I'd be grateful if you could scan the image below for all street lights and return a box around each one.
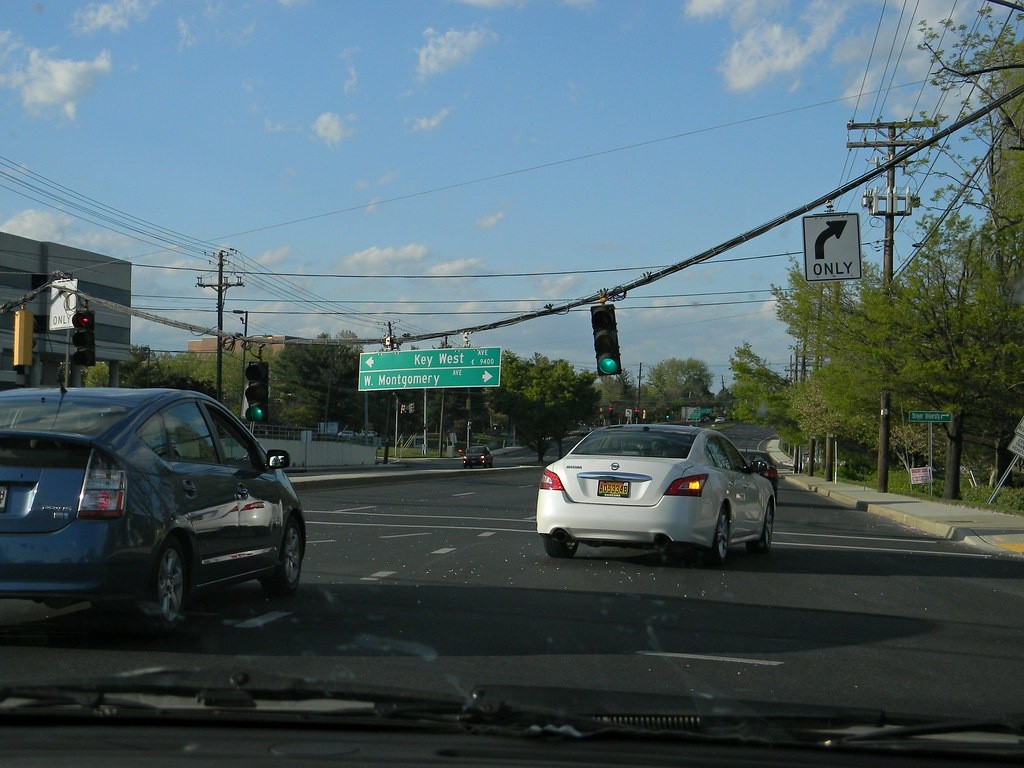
[233,308,249,420]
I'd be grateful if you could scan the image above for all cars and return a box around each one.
[463,446,493,468]
[536,423,776,564]
[0,387,307,635]
[714,417,725,423]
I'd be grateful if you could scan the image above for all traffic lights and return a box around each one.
[246,360,268,422]
[665,412,669,421]
[590,304,622,377]
[71,308,96,365]
[608,407,612,417]
[635,409,638,417]
